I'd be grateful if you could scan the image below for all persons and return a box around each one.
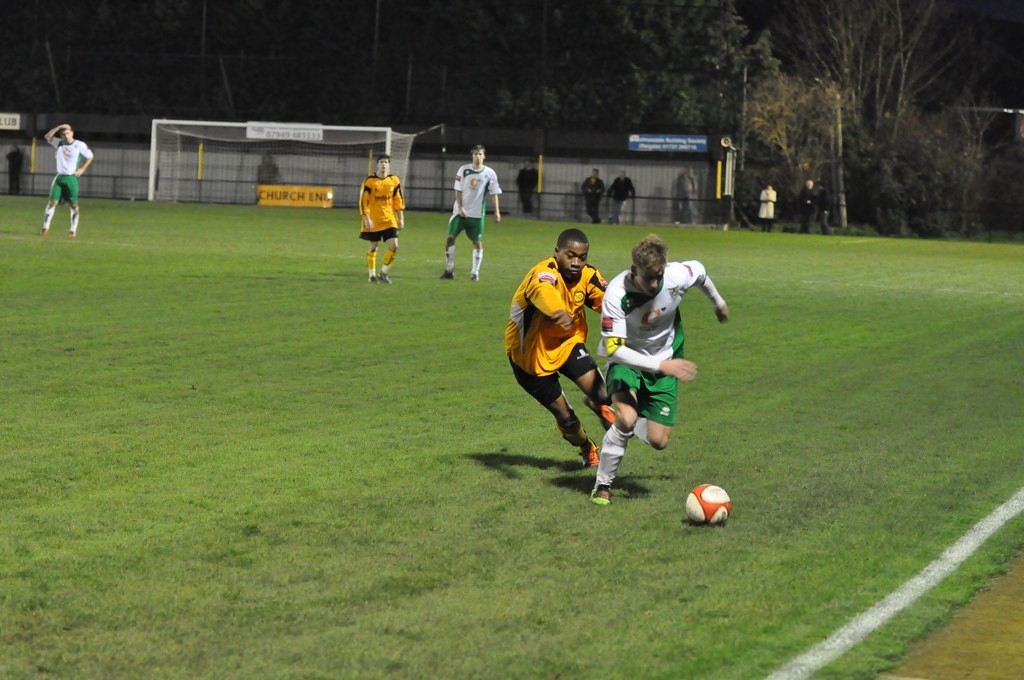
[40,123,94,238]
[581,168,606,224]
[358,153,406,285]
[758,182,777,233]
[439,145,503,282]
[798,179,818,235]
[516,158,539,220]
[588,232,730,507]
[815,182,836,235]
[254,151,279,204]
[6,143,25,196]
[605,170,636,225]
[504,228,618,469]
[671,168,697,226]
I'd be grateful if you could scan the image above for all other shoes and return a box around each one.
[369,276,378,283]
[379,272,392,284]
[40,229,48,236]
[68,231,76,237]
[440,270,455,280]
[470,274,479,281]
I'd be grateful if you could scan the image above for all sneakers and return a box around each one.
[578,437,600,468]
[584,395,617,429]
[589,484,611,505]
[600,419,634,439]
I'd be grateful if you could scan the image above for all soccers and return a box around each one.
[685,483,733,525]
